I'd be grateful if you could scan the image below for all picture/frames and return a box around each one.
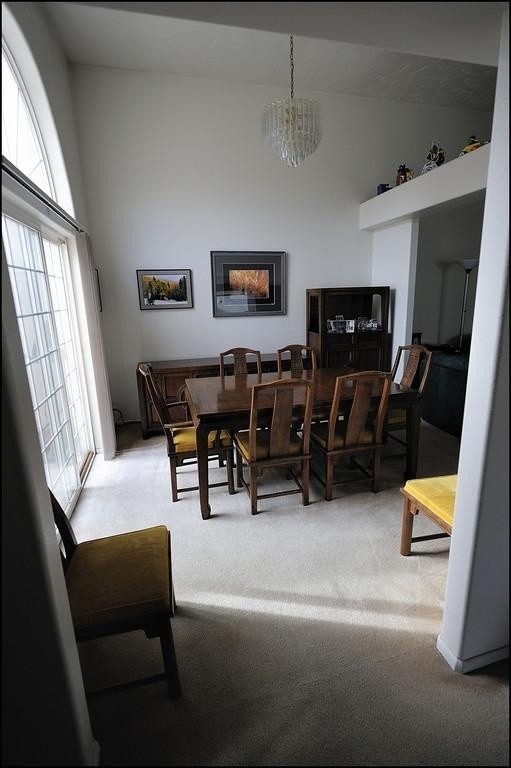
[210,251,288,318]
[95,267,103,311]
[136,269,193,311]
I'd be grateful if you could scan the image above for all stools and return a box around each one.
[399,474,457,556]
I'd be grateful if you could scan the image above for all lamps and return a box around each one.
[262,33,322,170]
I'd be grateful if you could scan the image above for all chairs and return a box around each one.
[46,490,179,703]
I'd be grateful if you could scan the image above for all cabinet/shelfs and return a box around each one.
[306,287,390,370]
